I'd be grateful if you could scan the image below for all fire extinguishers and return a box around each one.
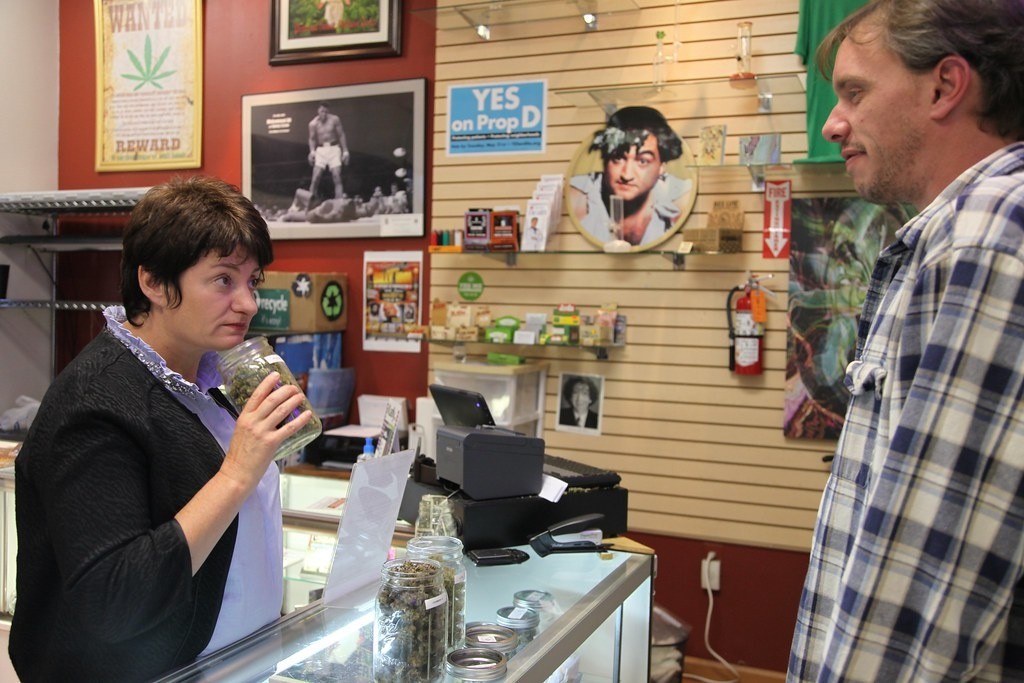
[725,273,776,377]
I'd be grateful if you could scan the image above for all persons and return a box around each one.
[784,0,1024,683]
[571,106,690,246]
[560,377,597,427]
[8,178,313,682]
[280,104,412,223]
[527,217,543,247]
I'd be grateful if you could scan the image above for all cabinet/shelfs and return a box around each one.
[429,248,685,361]
[0,440,658,683]
[0,184,152,440]
[434,358,551,440]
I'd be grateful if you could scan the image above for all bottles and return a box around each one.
[372,556,449,683]
[440,589,555,683]
[405,535,467,654]
[215,335,322,462]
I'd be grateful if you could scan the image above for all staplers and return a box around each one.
[529,514,606,558]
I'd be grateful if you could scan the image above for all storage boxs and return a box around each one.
[682,210,746,254]
[429,301,626,345]
[246,271,347,387]
[428,174,565,254]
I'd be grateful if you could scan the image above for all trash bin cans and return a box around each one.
[650,602,692,683]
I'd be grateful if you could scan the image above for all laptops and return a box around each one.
[429,382,622,491]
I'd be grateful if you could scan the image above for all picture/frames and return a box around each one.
[239,76,427,241]
[269,0,403,67]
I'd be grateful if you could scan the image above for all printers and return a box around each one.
[435,424,546,502]
